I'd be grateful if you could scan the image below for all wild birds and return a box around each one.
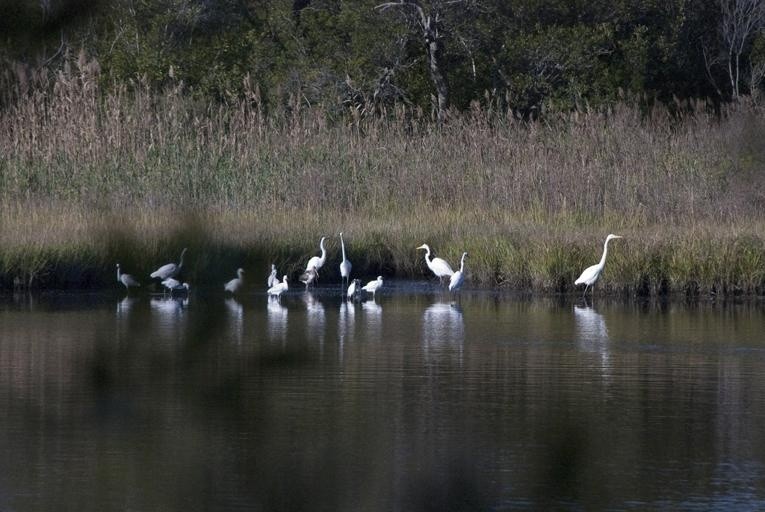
[115,263,141,291]
[297,235,330,292]
[339,274,384,300]
[447,251,470,296]
[223,267,246,296]
[415,243,455,282]
[573,233,624,298]
[337,231,354,284]
[266,262,290,298]
[149,247,194,294]
